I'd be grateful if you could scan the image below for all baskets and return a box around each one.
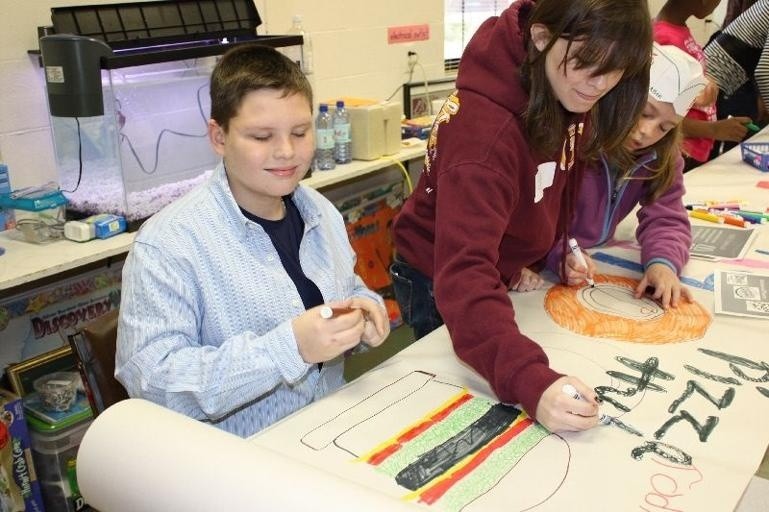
[741,143,769,172]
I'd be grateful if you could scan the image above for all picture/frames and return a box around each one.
[8,345,79,401]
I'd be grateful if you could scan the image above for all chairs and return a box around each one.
[68,306,134,418]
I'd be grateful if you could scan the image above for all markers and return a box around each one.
[568,238,597,291]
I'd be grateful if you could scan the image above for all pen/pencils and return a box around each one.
[565,386,585,403]
[321,308,356,320]
[746,155,769,170]
[727,114,759,133]
[685,199,769,228]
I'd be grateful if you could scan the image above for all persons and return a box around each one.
[544,42,710,313]
[390,1,653,434]
[649,0,769,173]
[114,44,390,440]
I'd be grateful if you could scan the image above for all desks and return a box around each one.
[248,127,768,512]
[0,127,428,293]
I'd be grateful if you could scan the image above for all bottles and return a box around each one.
[64,214,126,242]
[315,102,352,170]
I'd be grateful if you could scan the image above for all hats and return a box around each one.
[648,41,710,118]
